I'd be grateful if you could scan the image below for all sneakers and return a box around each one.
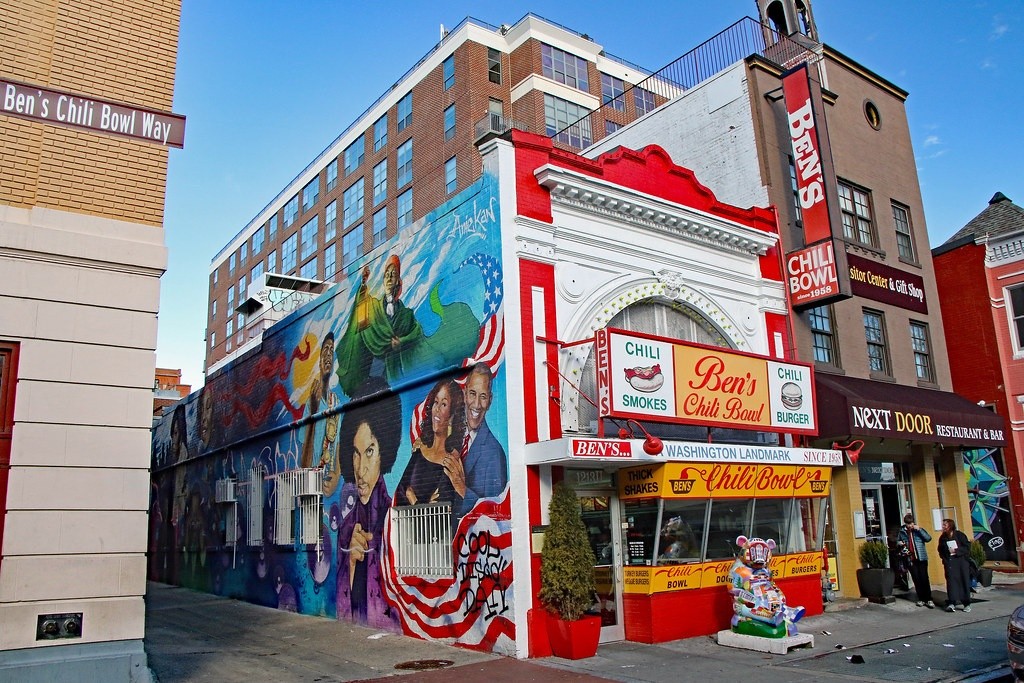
[946,604,956,612]
[963,605,971,612]
[916,601,925,607]
[926,600,936,608]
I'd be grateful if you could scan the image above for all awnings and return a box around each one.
[810,372,1008,447]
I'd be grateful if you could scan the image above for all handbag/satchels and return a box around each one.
[902,553,913,568]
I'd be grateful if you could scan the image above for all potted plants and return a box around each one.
[537,481,601,660]
[969,539,993,586]
[856,541,895,597]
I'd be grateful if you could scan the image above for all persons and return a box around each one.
[937,519,972,613]
[896,515,936,608]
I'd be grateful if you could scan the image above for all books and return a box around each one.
[947,540,958,555]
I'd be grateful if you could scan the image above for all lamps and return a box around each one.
[906,440,913,448]
[618,419,663,455]
[933,442,940,449]
[879,438,885,445]
[833,440,864,466]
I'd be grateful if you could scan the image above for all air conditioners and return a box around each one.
[296,471,316,494]
[216,479,236,501]
